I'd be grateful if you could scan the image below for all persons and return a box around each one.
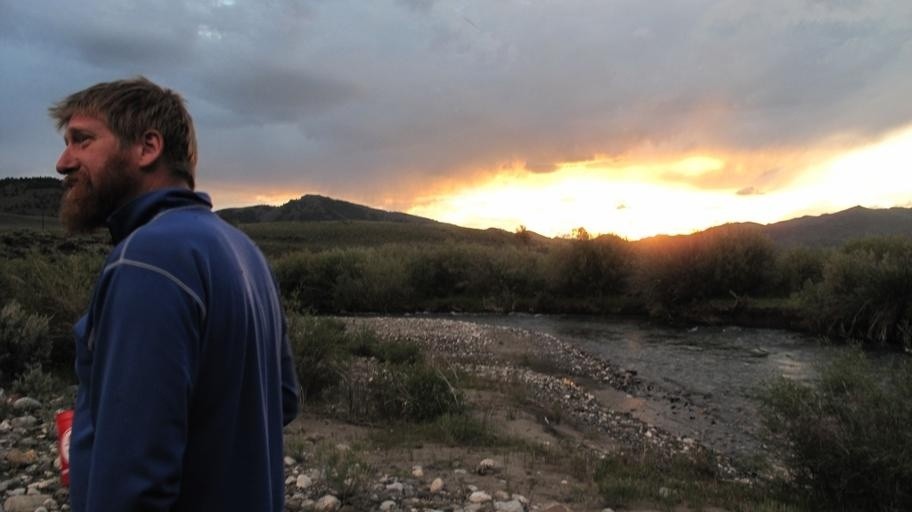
[47,75,302,511]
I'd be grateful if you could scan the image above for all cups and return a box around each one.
[55,410,76,486]
